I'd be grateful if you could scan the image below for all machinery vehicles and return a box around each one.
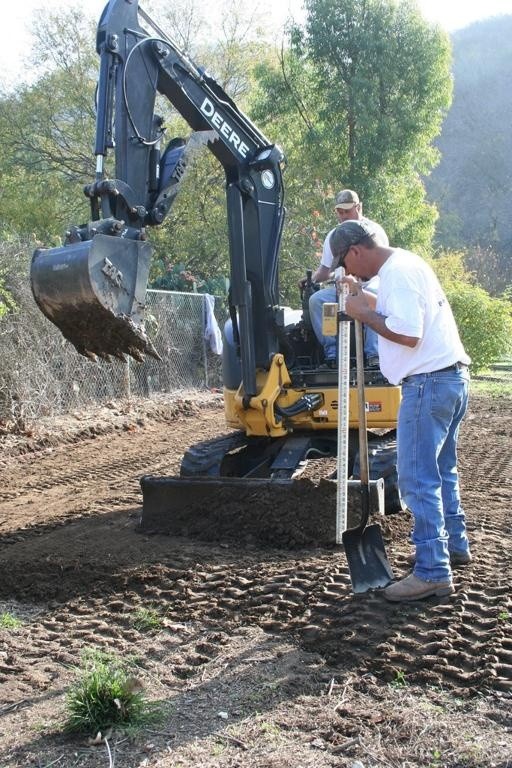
[30,0,407,526]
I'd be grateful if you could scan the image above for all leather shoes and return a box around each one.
[407,548,473,565]
[381,572,456,601]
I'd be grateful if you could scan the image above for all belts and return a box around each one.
[438,361,469,372]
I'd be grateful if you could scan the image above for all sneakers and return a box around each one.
[318,357,380,370]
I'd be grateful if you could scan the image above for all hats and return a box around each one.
[334,188,359,210]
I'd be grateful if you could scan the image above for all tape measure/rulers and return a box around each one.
[337,277,348,546]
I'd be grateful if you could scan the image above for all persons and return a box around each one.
[296,189,390,371]
[327,221,473,605]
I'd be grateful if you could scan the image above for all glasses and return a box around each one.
[338,248,351,268]
[328,220,376,273]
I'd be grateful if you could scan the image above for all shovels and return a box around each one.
[343,320,396,595]
